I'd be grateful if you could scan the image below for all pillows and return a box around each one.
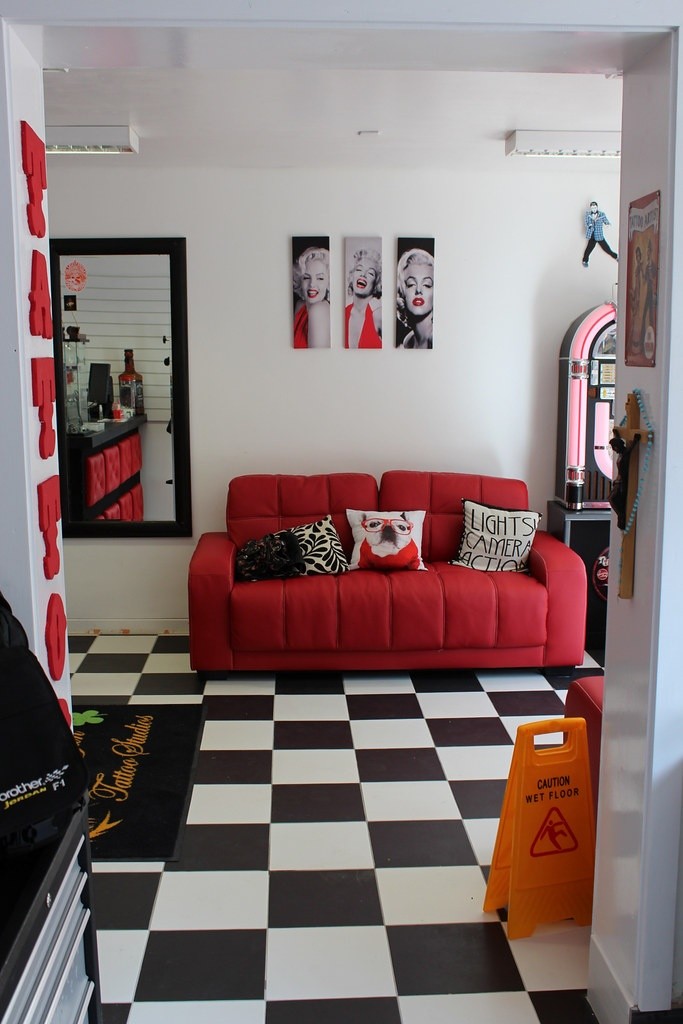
[447,497,543,573]
[273,513,351,576]
[345,508,430,571]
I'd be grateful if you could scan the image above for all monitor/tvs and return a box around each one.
[87,363,114,420]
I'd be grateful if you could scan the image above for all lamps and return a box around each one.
[44,125,140,154]
[505,130,621,159]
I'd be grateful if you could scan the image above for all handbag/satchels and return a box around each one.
[234,531,307,581]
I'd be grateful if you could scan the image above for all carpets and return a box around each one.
[72,703,209,862]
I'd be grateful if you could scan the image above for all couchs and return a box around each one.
[187,468,587,679]
[563,675,604,822]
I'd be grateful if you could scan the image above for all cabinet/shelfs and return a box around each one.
[547,499,612,666]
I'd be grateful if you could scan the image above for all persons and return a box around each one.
[293,247,331,348]
[396,249,433,349]
[345,250,382,349]
[609,429,640,530]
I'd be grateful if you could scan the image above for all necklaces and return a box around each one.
[620,388,653,533]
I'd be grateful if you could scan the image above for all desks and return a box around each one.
[66,414,148,521]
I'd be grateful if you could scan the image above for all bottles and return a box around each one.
[119,348,144,416]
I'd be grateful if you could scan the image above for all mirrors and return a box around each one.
[50,236,194,539]
[554,303,617,510]
